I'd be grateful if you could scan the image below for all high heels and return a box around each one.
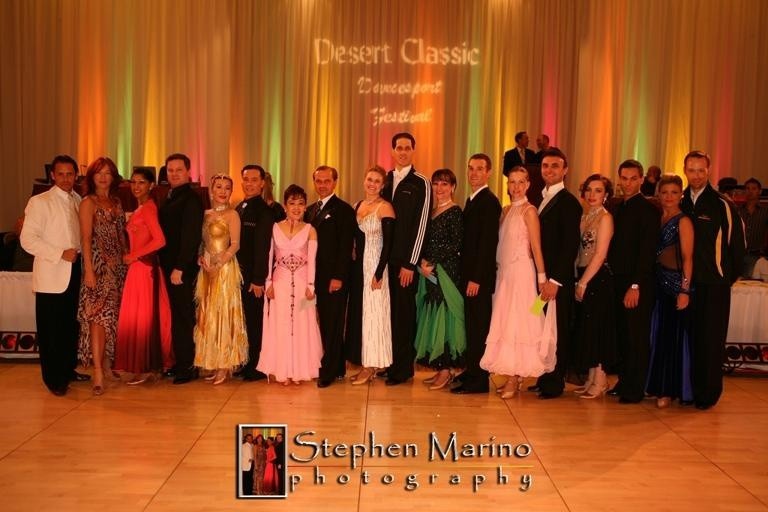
[203,373,216,382]
[344,365,365,381]
[91,384,105,396]
[423,376,438,384]
[351,369,375,385]
[496,376,523,394]
[577,381,610,399]
[124,372,157,386]
[212,367,233,386]
[428,374,452,391]
[102,368,122,382]
[572,381,593,394]
[500,379,519,400]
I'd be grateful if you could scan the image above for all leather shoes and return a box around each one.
[70,370,91,382]
[695,402,709,410]
[606,384,625,396]
[173,374,192,384]
[317,379,332,388]
[679,399,693,406]
[451,373,473,384]
[527,385,540,392]
[242,373,267,382]
[536,386,565,400]
[618,389,644,404]
[51,386,67,396]
[385,370,416,386]
[376,369,395,377]
[450,377,490,393]
[161,368,177,378]
[231,367,252,378]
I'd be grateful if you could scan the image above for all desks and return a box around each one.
[725,281,768,379]
[1,271,41,358]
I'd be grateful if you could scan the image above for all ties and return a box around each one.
[310,200,323,220]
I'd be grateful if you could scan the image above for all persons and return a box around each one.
[240,428,285,496]
[20,130,767,410]
